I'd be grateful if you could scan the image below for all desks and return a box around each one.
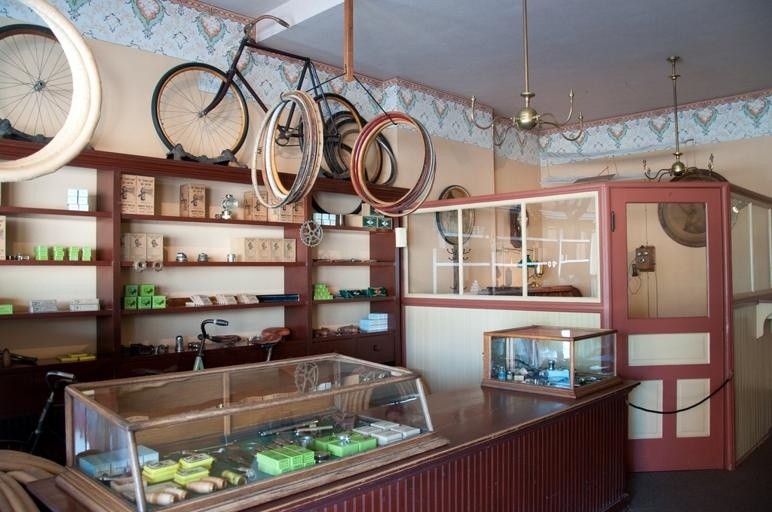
[23,367,640,512]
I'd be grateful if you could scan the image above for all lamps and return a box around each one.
[469,1,584,154]
[640,55,717,181]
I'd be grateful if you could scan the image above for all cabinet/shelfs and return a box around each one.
[0,139,403,450]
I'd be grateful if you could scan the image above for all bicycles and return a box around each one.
[149,13,385,189]
[0,370,75,456]
[1,23,73,142]
[190,317,283,373]
